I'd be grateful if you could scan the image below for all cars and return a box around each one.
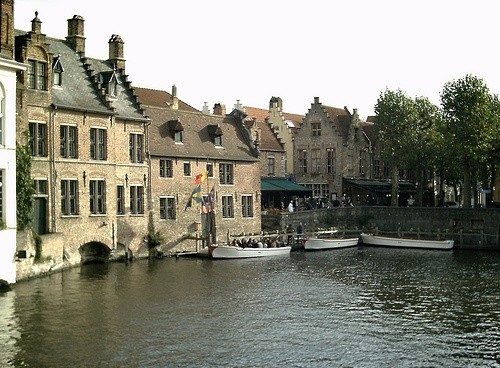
[436,201,462,209]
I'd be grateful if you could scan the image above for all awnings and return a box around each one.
[349,178,427,194]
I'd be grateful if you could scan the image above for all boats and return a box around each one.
[207,242,293,260]
[360,233,454,251]
[299,236,358,251]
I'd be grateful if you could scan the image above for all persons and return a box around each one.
[231,237,285,248]
[341,197,354,207]
[287,222,302,246]
[280,199,299,213]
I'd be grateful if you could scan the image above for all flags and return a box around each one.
[185,173,214,213]
